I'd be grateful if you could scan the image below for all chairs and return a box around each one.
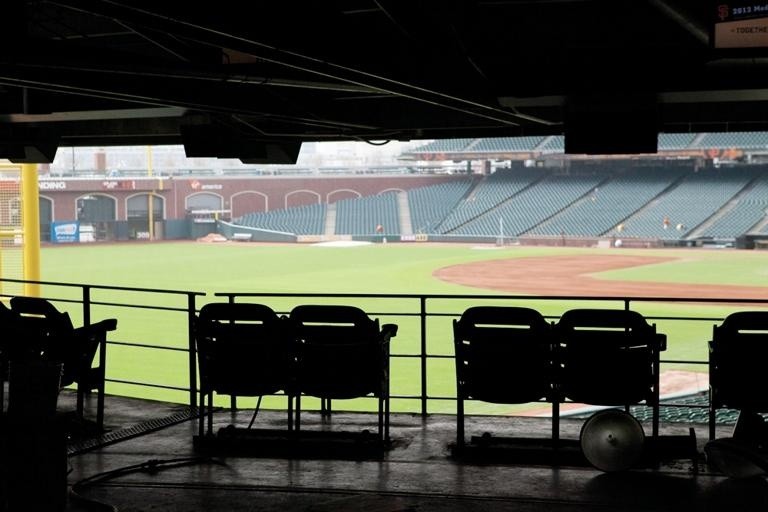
[657,132,767,150]
[334,192,401,235]
[231,203,327,235]
[397,136,564,175]
[0,296,118,460]
[192,302,398,459]
[453,306,668,465]
[406,169,768,248]
[704,311,768,465]
